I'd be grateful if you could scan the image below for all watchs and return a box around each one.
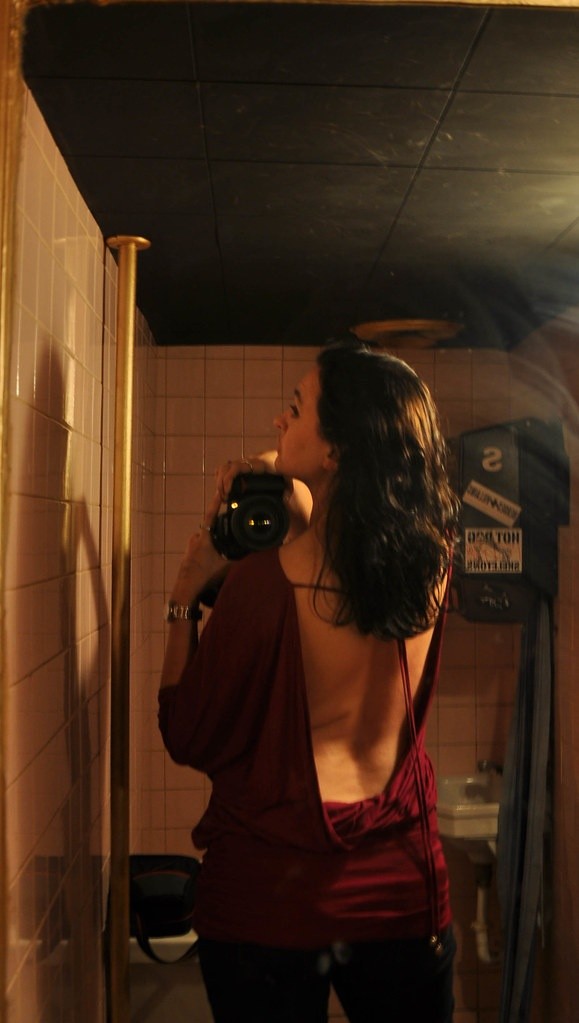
[167,600,203,622]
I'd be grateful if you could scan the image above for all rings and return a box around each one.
[197,523,213,532]
[242,460,254,474]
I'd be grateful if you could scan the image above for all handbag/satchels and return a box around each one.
[19,856,67,963]
[130,854,203,964]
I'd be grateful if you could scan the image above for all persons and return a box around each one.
[158,350,458,1023]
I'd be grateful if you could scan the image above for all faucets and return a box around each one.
[477,759,504,775]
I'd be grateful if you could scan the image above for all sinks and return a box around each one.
[429,774,503,841]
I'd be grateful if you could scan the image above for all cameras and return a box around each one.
[209,471,294,560]
